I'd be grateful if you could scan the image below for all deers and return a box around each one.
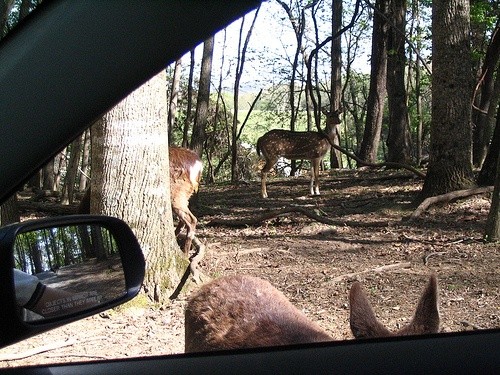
[256,107,342,199]
[184,273,442,354]
[63,146,205,258]
